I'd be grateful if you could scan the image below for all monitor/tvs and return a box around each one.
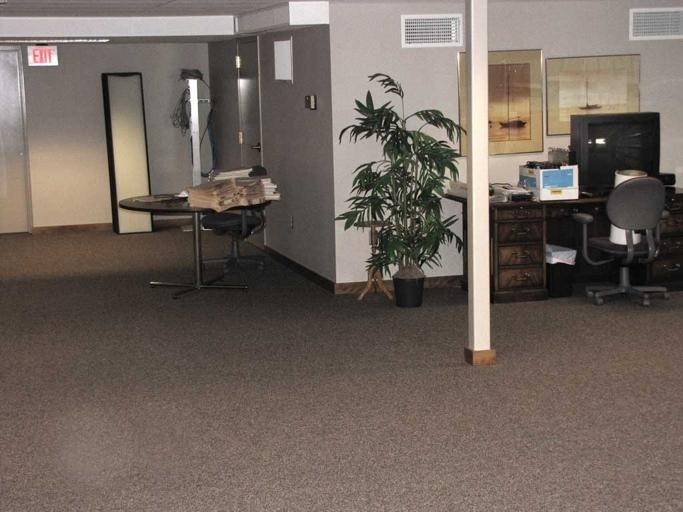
[570,112,660,189]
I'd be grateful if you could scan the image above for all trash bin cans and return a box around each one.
[546,245,577,297]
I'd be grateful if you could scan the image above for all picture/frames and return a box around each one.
[456,48,543,158]
[544,53,642,136]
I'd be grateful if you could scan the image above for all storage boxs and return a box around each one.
[518,163,580,202]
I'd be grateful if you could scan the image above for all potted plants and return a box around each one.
[332,68,466,308]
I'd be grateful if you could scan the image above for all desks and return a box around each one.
[118,192,273,300]
[353,220,396,301]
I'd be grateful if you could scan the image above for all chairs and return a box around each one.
[200,165,268,275]
[568,174,671,308]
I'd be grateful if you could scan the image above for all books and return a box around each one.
[186,167,281,213]
[136,193,178,202]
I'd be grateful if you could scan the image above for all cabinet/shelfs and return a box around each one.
[444,182,682,302]
